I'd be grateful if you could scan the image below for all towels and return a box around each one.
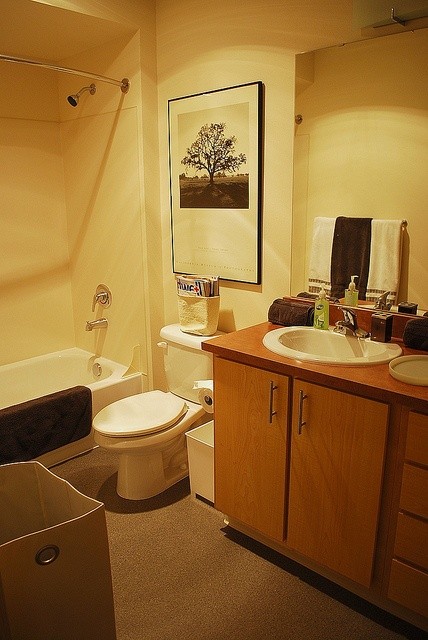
[268,298,315,326]
[308,217,336,298]
[295,291,317,298]
[331,217,372,301]
[365,218,405,305]
[423,310,428,315]
[402,319,428,353]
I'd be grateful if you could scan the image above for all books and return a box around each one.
[176,274,220,298]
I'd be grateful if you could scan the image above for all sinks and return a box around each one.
[262,325,403,366]
[360,304,428,316]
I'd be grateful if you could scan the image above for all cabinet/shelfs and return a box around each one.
[384,407,427,640]
[201,325,391,592]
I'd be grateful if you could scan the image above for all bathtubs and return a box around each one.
[0,347,142,468]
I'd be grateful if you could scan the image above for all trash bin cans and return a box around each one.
[185,420,215,503]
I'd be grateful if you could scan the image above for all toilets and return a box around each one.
[92,323,227,502]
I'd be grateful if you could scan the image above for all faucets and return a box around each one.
[374,291,393,311]
[85,318,107,332]
[333,307,369,339]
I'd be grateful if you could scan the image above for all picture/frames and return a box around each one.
[167,82,262,285]
